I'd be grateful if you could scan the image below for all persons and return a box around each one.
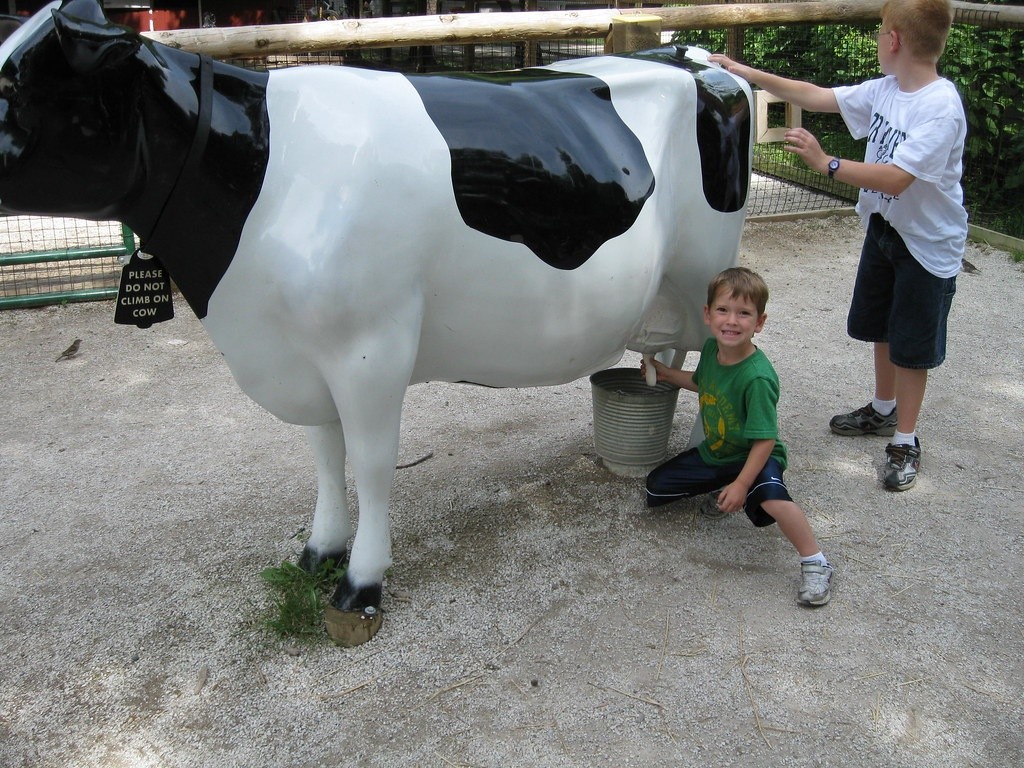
[706,0,968,491]
[641,267,833,607]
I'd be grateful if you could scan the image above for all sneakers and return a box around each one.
[796,559,836,605]
[829,402,897,436]
[882,436,922,491]
[700,487,733,520]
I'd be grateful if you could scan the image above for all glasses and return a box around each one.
[873,31,904,47]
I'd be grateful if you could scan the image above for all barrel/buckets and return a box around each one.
[588,367,679,467]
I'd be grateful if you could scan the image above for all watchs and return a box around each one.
[827,156,841,179]
